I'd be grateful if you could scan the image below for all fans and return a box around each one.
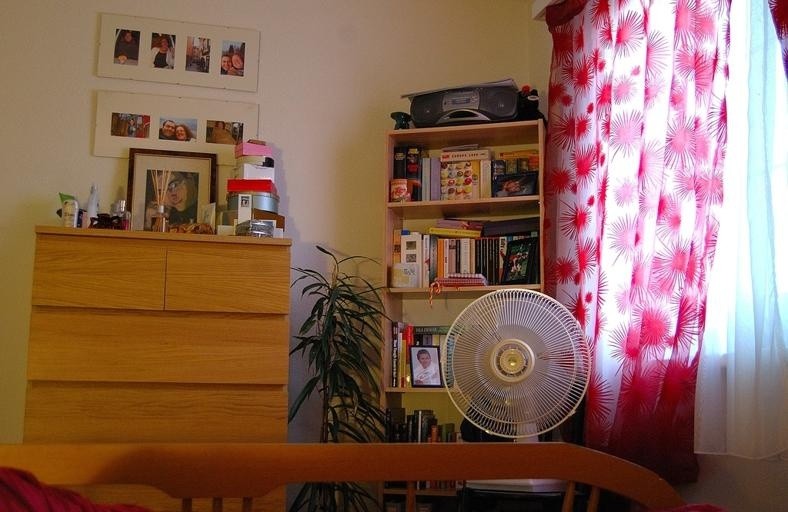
[439,288,595,496]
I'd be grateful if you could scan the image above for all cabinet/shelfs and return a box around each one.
[378,119,550,508]
[15,217,296,509]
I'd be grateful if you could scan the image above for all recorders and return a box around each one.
[409,86,518,128]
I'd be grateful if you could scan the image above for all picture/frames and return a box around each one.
[406,343,443,388]
[122,145,221,235]
[497,235,540,287]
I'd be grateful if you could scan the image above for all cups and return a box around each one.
[390,179,411,202]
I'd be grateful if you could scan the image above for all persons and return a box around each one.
[151,36,174,70]
[229,51,244,77]
[211,121,237,145]
[175,124,196,142]
[113,27,138,67]
[126,118,142,138]
[158,119,175,141]
[220,54,231,74]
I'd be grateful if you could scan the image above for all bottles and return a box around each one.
[61,184,131,230]
